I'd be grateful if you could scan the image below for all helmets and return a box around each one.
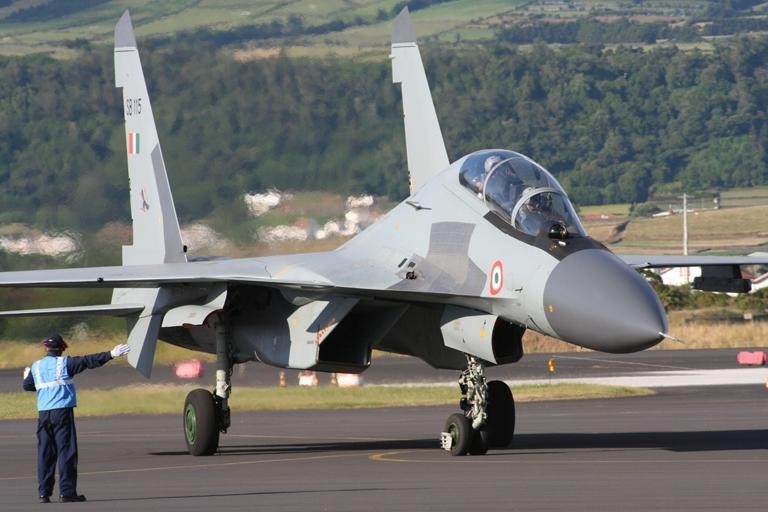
[523,187,541,205]
[484,156,505,173]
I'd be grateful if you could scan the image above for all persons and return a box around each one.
[514,188,563,235]
[20,332,133,503]
[471,155,525,206]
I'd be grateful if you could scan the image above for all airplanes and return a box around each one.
[0,2,768,458]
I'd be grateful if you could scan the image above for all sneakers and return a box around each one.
[59,493,86,503]
[39,495,51,503]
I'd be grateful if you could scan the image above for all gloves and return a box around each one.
[111,343,130,358]
[23,367,31,380]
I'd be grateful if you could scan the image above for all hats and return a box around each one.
[42,333,68,350]
